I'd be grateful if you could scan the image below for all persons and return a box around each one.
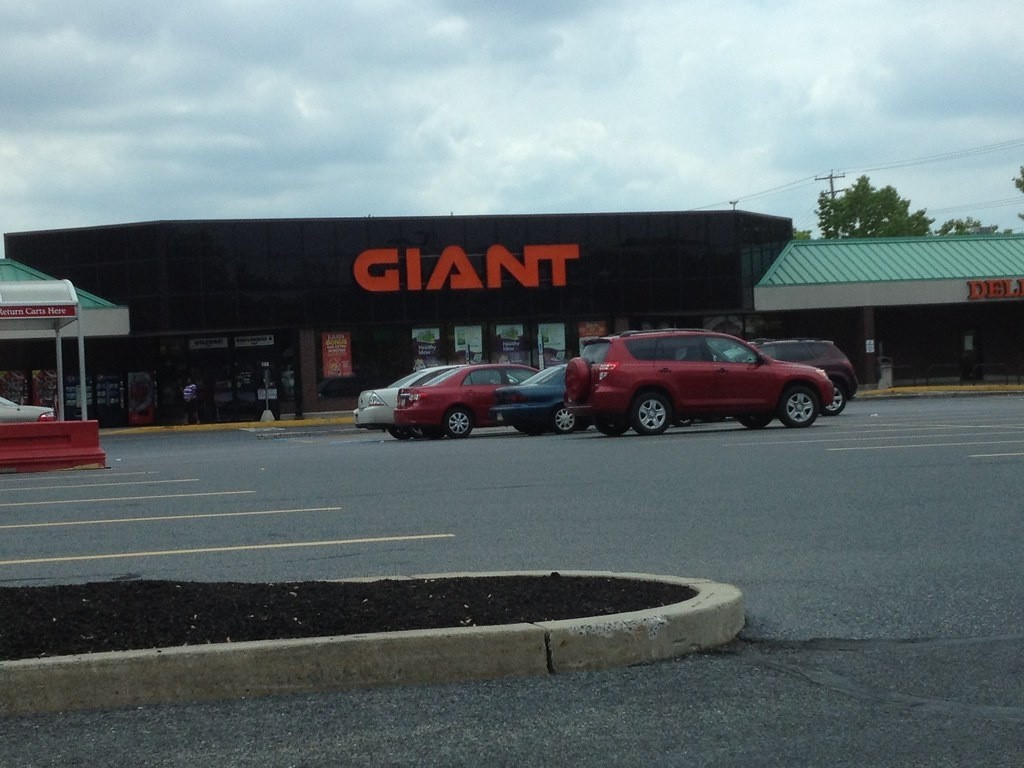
[182,377,201,424]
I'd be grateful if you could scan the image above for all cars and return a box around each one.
[722,338,858,417]
[487,365,695,435]
[352,365,522,440]
[0,397,55,422]
[394,363,546,438]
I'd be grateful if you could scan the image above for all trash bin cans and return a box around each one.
[878,357,893,387]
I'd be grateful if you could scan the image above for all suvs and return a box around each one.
[563,329,835,436]
